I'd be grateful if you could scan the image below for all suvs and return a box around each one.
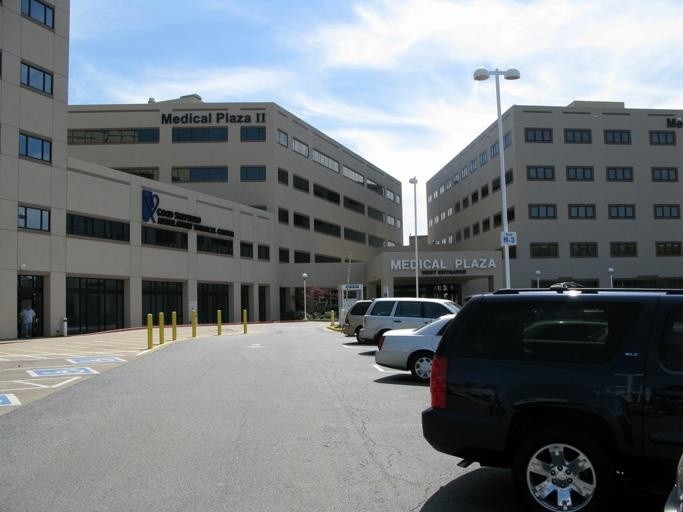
[359,297,463,350]
[419,286,683,511]
[343,299,373,344]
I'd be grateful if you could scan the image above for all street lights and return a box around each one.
[301,273,308,320]
[606,267,615,287]
[473,66,521,289]
[535,270,540,288]
[409,176,419,300]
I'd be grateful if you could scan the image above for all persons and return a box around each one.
[18,303,36,338]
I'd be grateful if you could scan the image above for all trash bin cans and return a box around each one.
[62,316,68,337]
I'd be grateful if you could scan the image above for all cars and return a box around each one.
[374,314,456,384]
